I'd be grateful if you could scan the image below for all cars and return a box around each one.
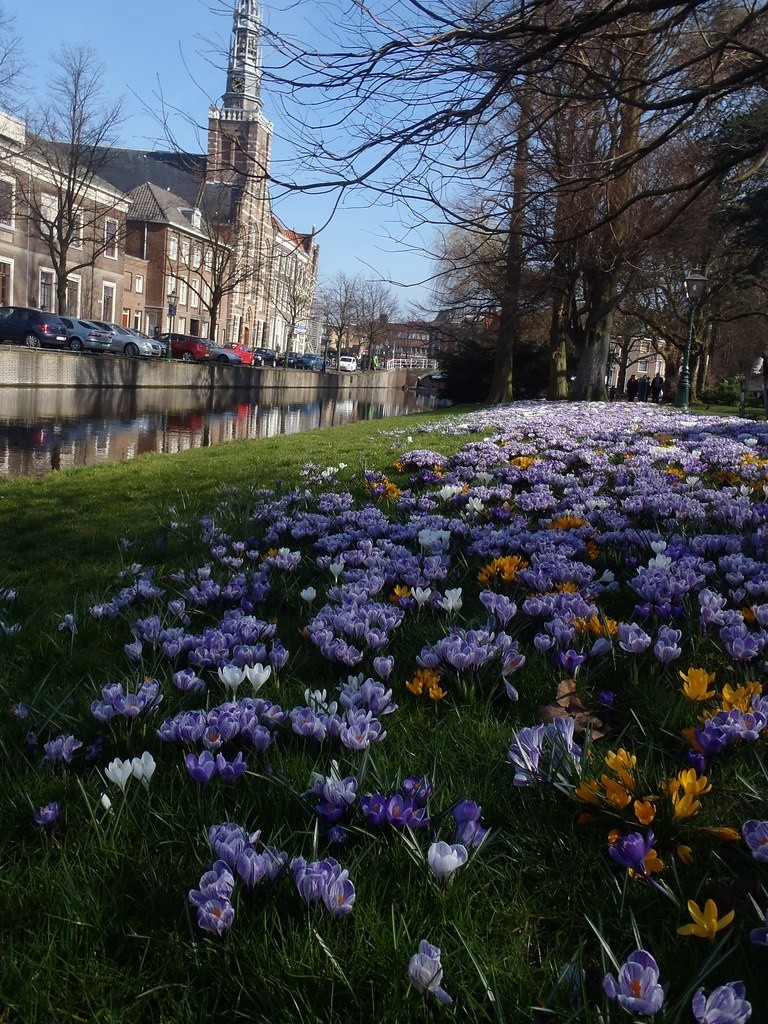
[58,316,113,353]
[250,347,277,368]
[207,340,243,364]
[276,351,306,370]
[80,318,162,358]
[336,356,356,372]
[122,327,167,357]
[0,306,69,348]
[223,342,256,366]
[360,354,381,371]
[150,333,211,361]
[301,353,322,371]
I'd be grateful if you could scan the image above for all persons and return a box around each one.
[30,297,49,312]
[625,372,664,405]
[275,343,281,354]
